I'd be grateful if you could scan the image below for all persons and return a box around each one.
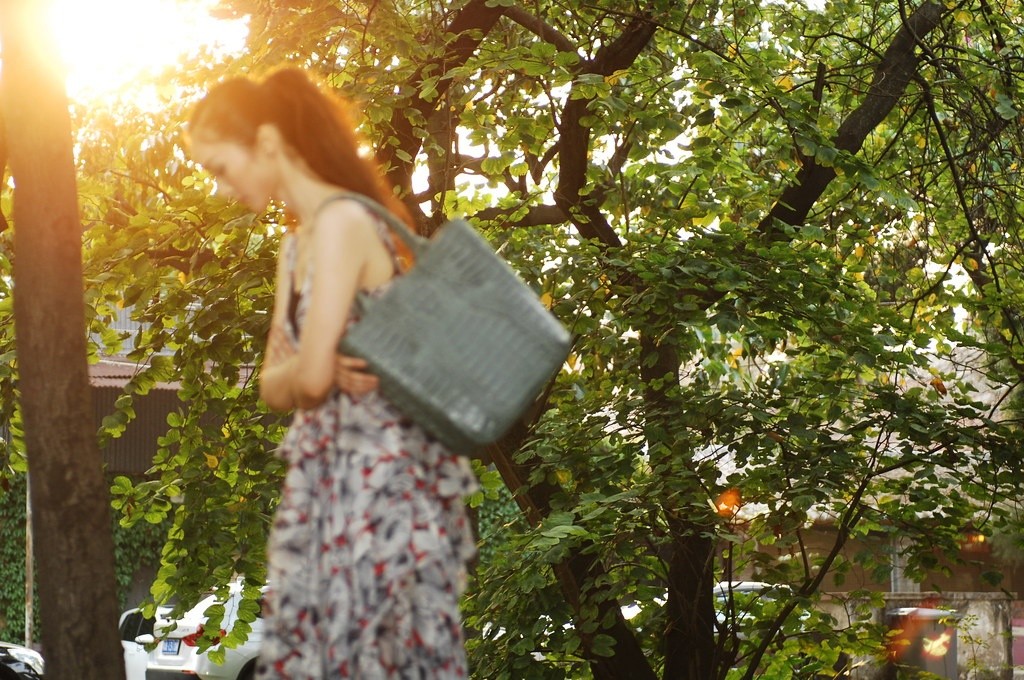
[186,65,470,679]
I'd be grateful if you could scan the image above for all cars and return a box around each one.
[0,641,45,680]
[147,572,264,680]
[118,605,177,680]
[482,578,811,666]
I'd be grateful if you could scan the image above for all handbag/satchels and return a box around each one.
[309,191,571,454]
